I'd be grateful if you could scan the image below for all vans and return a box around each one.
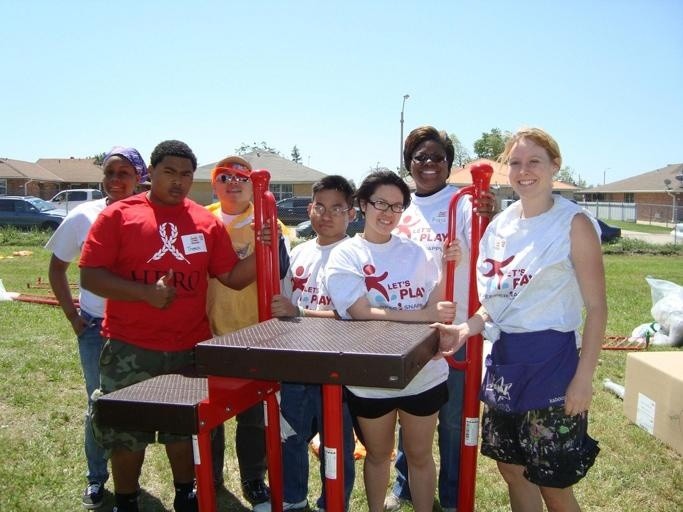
[44,189,103,213]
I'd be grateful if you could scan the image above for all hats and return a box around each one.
[215,154,252,171]
[212,162,251,178]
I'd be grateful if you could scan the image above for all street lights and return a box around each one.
[603,166,611,184]
[398,93,411,175]
[664,171,683,246]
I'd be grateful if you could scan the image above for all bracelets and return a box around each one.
[297,306,304,319]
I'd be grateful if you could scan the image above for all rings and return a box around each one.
[491,205,495,212]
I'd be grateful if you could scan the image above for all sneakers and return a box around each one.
[383,493,401,512]
[242,477,268,503]
[174,480,197,512]
[253,499,307,512]
[112,493,138,512]
[82,482,103,510]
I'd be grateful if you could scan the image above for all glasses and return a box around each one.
[311,202,350,217]
[410,153,446,163]
[367,198,405,213]
[214,173,250,183]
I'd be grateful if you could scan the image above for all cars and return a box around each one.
[597,217,623,245]
[294,207,366,240]
[0,195,69,231]
[670,223,683,244]
[272,196,363,225]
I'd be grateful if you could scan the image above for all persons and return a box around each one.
[43,145,149,510]
[253,175,355,512]
[202,157,291,503]
[323,170,461,512]
[430,127,607,512]
[383,124,502,512]
[78,140,281,512]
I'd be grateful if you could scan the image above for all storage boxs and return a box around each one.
[621,344,682,459]
